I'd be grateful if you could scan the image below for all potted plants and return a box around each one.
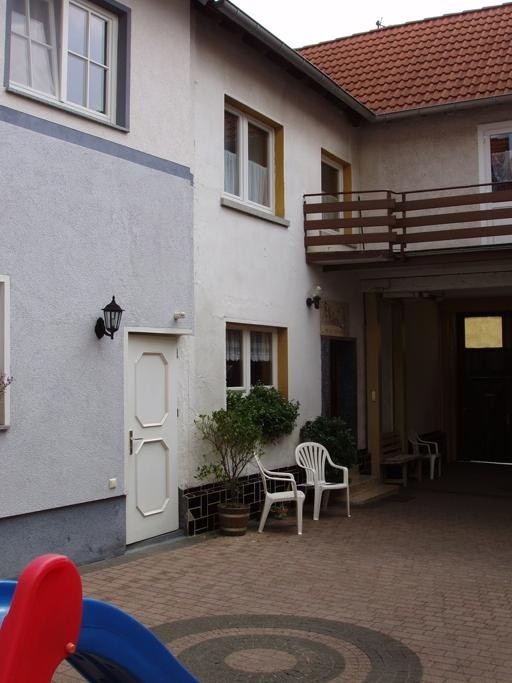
[194,377,301,535]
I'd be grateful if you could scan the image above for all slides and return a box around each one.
[0,556,194,683]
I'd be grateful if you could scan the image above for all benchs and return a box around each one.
[381,431,421,487]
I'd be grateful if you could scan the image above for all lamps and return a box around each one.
[306,282,324,312]
[94,293,124,342]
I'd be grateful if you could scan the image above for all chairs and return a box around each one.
[252,451,307,537]
[405,429,443,480]
[295,440,352,521]
